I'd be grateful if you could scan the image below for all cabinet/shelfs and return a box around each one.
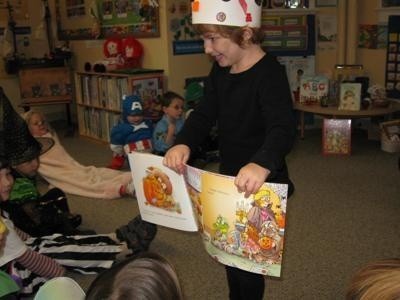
[72,69,168,147]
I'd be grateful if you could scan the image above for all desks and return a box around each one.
[293,96,400,141]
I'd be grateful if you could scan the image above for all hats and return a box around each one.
[123,95,143,116]
[191,0,262,29]
[0,86,55,169]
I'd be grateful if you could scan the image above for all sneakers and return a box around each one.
[62,214,81,229]
[117,214,157,254]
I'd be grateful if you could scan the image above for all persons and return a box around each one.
[346,256,400,300]
